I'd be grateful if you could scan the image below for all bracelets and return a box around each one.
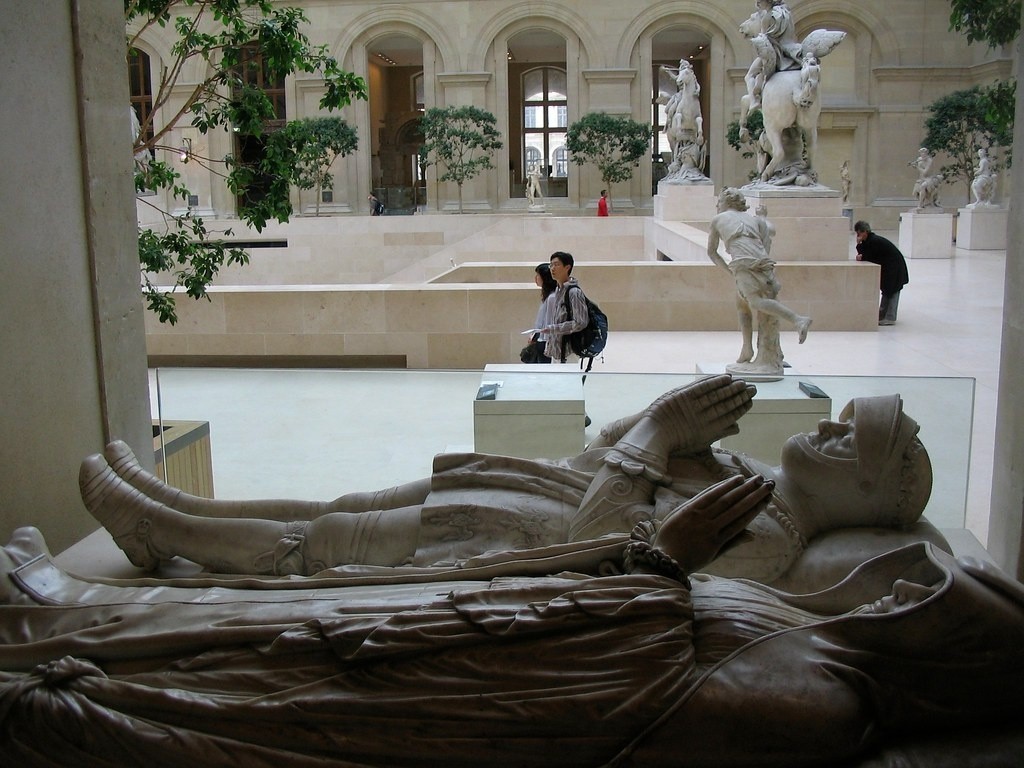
[624,518,694,590]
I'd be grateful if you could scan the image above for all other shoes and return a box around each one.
[585,416,591,428]
[879,320,895,326]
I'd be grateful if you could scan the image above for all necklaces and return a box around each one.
[704,456,804,559]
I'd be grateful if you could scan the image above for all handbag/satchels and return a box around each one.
[519,346,536,363]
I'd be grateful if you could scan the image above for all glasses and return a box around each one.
[546,262,565,268]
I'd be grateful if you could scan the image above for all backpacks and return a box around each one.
[564,284,609,359]
[374,200,385,215]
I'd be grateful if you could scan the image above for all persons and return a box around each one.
[368,191,381,216]
[0,471,1023,768]
[529,261,558,364]
[854,220,909,326]
[662,61,700,134]
[840,161,853,203]
[707,185,813,361]
[527,164,547,207]
[744,0,803,117]
[77,373,935,585]
[907,147,936,208]
[597,190,609,217]
[971,149,992,205]
[540,252,591,427]
